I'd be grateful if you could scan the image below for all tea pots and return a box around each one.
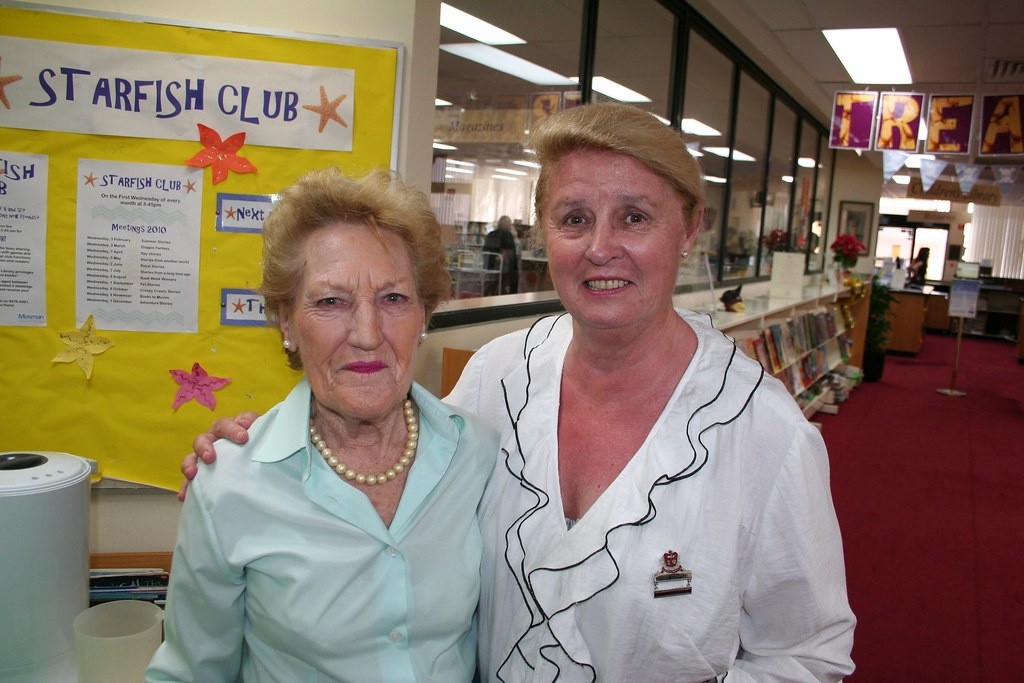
[1,451,98,683]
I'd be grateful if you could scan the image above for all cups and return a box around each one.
[72,600,166,683]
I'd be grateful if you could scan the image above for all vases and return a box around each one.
[837,270,851,282]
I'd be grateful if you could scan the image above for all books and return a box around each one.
[724,297,863,417]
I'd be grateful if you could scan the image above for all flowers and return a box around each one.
[830,234,866,270]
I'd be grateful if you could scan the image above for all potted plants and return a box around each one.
[859,276,900,381]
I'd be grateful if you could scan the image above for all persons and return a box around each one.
[176,98,858,682]
[906,247,931,288]
[143,164,499,683]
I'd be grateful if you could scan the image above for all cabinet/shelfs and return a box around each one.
[875,252,1024,363]
[686,274,870,431]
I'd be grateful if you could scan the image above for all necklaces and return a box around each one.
[304,394,420,485]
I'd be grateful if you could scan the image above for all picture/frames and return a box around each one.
[838,200,875,256]
